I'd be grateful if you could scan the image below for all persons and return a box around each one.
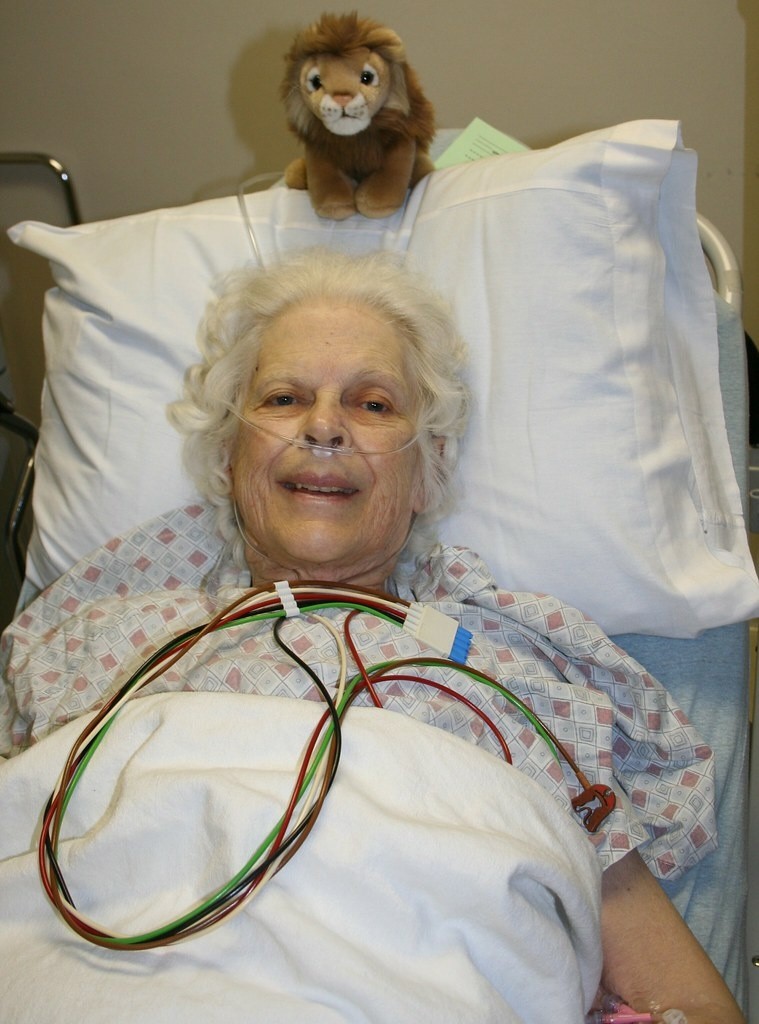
[0,253,744,1024]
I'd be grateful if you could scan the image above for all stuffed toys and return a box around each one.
[278,7,437,221]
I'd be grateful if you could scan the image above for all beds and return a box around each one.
[0,207,759,1024]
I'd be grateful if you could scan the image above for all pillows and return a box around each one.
[6,118,759,639]
